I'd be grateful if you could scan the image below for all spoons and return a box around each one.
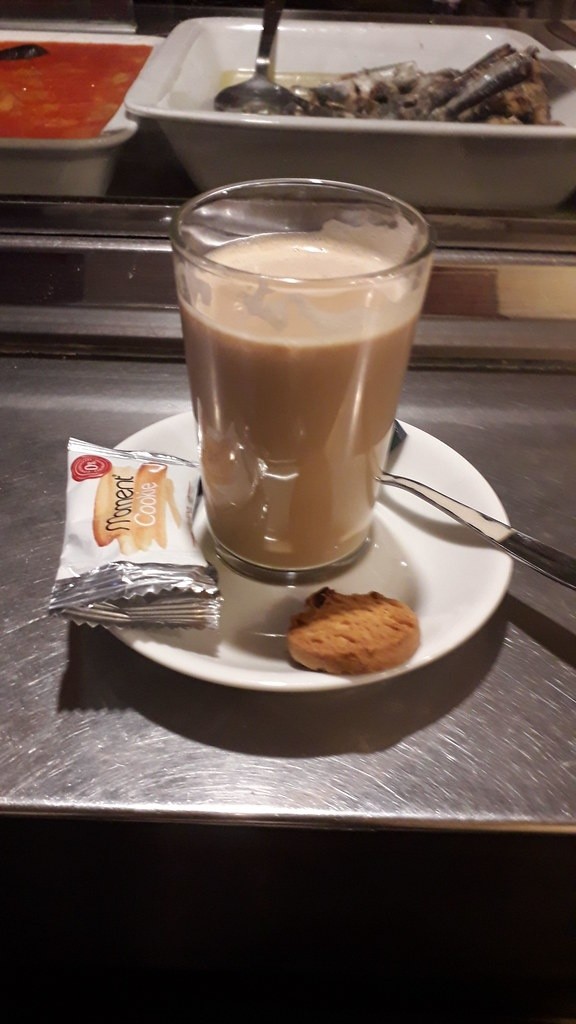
[213,0,296,114]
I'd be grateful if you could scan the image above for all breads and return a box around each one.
[92,462,169,555]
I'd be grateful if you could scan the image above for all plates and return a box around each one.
[104,408,516,691]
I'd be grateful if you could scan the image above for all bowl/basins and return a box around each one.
[0,31,164,199]
[121,14,575,211]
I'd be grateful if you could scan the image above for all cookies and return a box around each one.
[286,587,420,675]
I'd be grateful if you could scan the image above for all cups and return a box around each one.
[168,176,438,586]
